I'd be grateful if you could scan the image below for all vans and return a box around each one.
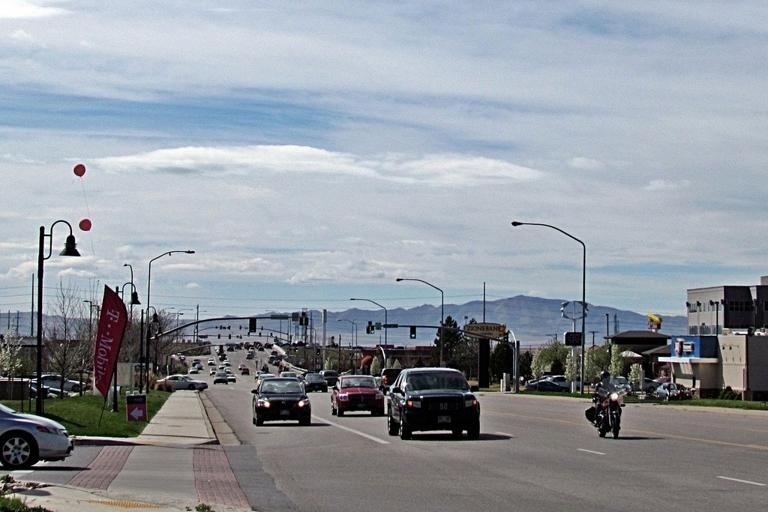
[380,368,405,394]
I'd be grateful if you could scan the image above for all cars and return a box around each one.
[154,341,340,393]
[0,373,89,400]
[330,375,385,416]
[250,377,314,427]
[0,404,76,469]
[524,374,693,400]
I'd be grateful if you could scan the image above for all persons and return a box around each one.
[584,369,622,428]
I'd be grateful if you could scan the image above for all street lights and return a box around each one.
[80,297,101,343]
[395,278,445,369]
[349,298,387,351]
[145,250,196,397]
[33,218,85,421]
[107,283,144,415]
[155,304,209,339]
[336,318,358,373]
[124,261,135,326]
[508,221,586,397]
[139,305,163,394]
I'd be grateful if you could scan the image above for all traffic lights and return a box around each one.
[408,327,417,339]
[366,323,376,334]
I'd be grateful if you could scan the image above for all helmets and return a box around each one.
[600,370,610,379]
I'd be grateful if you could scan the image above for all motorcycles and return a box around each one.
[588,381,625,439]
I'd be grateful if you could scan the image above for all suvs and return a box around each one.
[385,366,481,440]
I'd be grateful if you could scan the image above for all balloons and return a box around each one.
[78,218,91,232]
[73,164,85,177]
